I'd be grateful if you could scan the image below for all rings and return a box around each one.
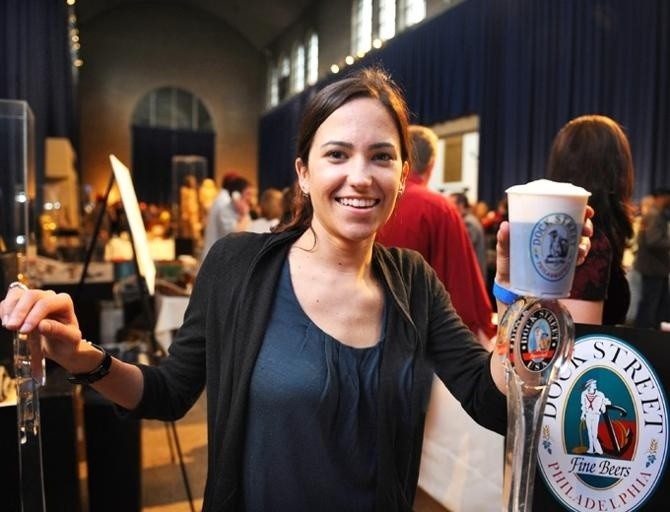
[9,281,26,289]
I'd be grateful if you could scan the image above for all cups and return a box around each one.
[505,185,593,300]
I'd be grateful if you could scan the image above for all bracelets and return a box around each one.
[492,282,518,305]
[66,341,112,385]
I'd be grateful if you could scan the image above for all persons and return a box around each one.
[450,193,507,290]
[2,73,594,511]
[545,115,668,339]
[201,171,302,263]
[376,124,500,347]
